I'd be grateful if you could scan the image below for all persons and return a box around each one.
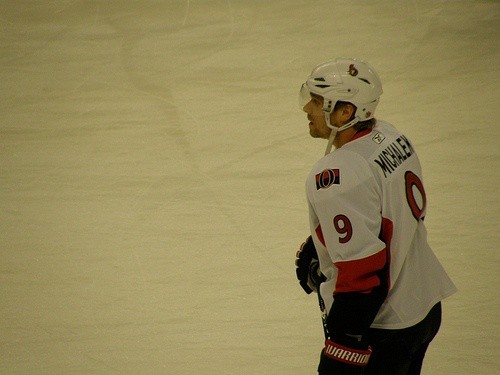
[294,57,460,375]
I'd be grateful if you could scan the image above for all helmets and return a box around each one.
[297,54,383,122]
